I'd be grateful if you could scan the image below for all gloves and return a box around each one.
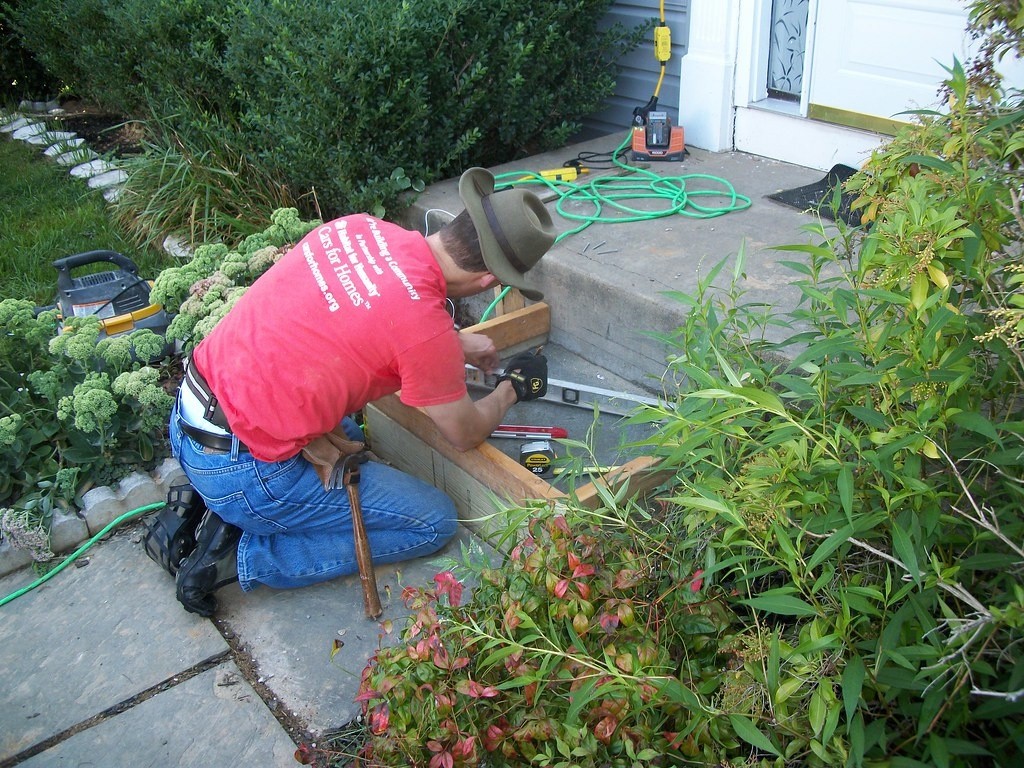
[495,349,548,404]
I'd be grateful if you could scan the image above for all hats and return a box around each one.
[459,167,556,301]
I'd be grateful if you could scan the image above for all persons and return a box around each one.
[141,166,558,617]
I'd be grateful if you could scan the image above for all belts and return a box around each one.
[176,381,249,452]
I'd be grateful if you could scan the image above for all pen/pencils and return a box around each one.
[534,345,544,356]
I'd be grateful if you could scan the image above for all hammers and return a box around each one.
[328,450,384,621]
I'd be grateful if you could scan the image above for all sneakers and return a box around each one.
[142,474,208,578]
[176,509,244,618]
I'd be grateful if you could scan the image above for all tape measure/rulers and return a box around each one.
[520,439,623,479]
